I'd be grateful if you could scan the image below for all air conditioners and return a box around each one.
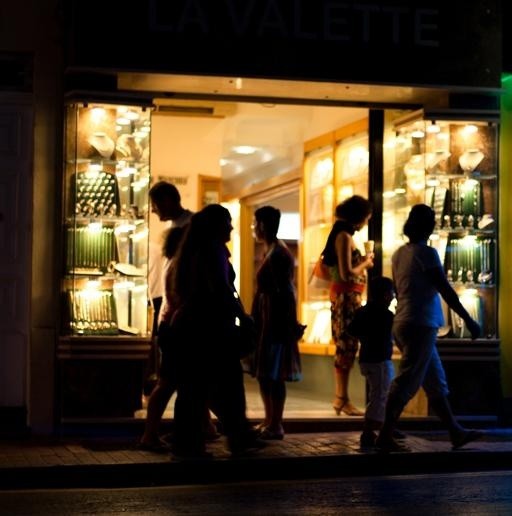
[116,71,239,118]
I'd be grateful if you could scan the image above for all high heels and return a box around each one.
[334,394,364,416]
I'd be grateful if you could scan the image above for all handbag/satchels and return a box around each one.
[315,257,336,280]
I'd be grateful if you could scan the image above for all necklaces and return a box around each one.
[70,137,121,330]
[409,151,492,334]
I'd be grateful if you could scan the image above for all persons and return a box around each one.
[358,276,406,448]
[376,203,480,453]
[324,195,375,416]
[136,227,185,455]
[176,204,257,456]
[149,179,197,346]
[250,206,302,441]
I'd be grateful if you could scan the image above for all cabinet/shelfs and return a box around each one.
[295,106,398,360]
[390,102,502,341]
[57,86,156,339]
